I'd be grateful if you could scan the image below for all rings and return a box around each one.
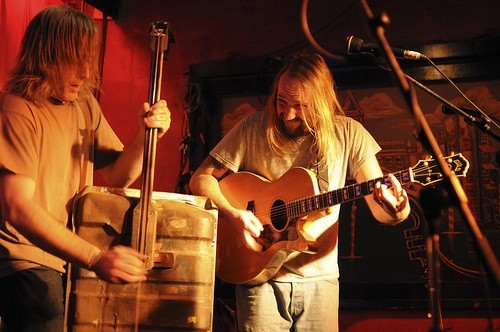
[379,200,385,203]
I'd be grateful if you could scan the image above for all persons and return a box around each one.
[189,53,410,332]
[0,6,171,332]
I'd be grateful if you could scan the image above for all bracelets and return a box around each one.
[90,250,104,271]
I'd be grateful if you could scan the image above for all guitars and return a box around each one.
[205,151,469,287]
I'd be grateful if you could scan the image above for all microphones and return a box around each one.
[442,104,484,119]
[345,35,428,60]
